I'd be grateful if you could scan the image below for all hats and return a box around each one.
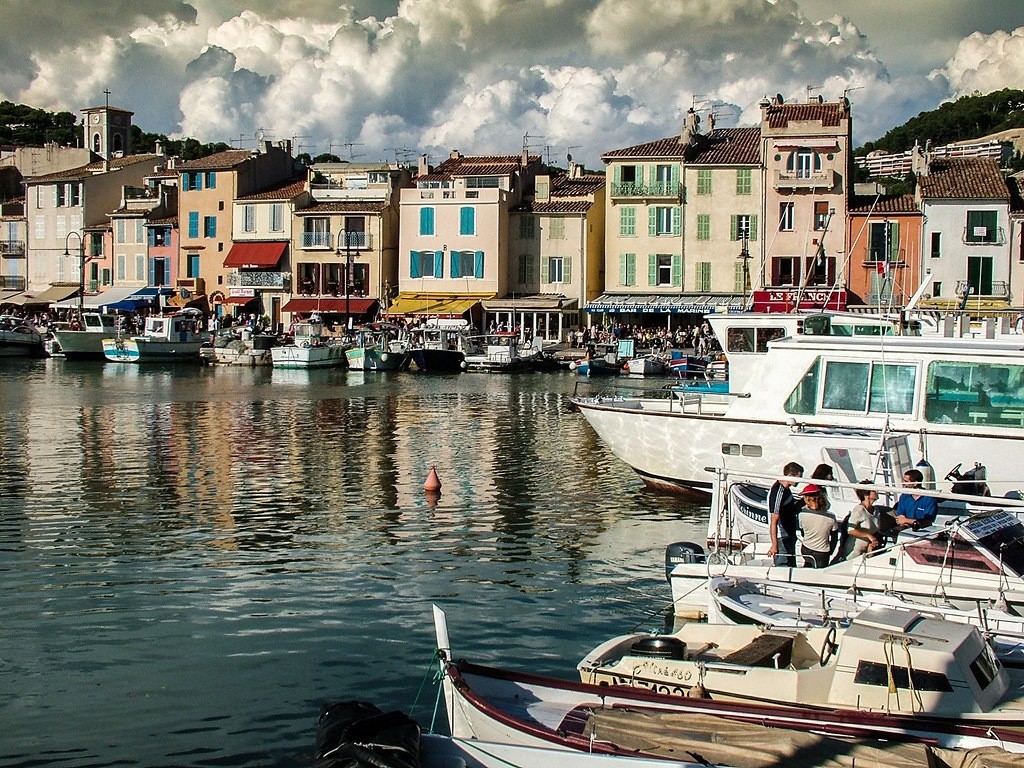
[798,484,823,495]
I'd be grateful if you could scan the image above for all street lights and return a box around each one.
[62,231,100,312]
[737,230,753,306]
[335,229,362,334]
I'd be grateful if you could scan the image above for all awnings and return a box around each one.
[223,242,289,268]
[0,286,207,309]
[223,297,259,306]
[382,294,496,318]
[281,299,377,313]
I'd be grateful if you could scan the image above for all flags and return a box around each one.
[877,261,891,275]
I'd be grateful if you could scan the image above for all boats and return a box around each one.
[51,312,139,359]
[434,274,1024,768]
[411,328,465,372]
[102,315,214,363]
[270,322,348,368]
[0,315,48,356]
[345,323,410,371]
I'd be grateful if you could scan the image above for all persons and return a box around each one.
[896,470,937,529]
[811,464,834,512]
[840,481,882,561]
[488,320,533,347]
[179,312,305,335]
[373,318,429,344]
[767,462,803,567]
[0,307,169,336]
[567,320,720,352]
[798,486,838,568]
